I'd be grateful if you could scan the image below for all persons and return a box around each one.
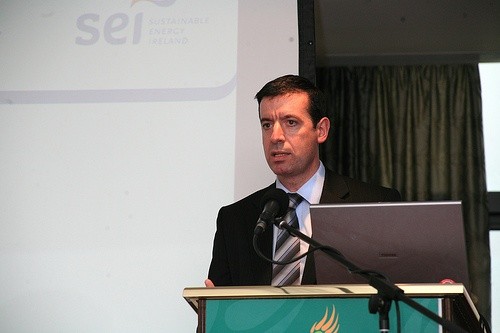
[204,74,404,288]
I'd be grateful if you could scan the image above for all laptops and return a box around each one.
[310,201,470,293]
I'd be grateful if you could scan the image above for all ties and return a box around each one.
[270,192,305,286]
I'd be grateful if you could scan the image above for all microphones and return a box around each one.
[254,188,288,235]
